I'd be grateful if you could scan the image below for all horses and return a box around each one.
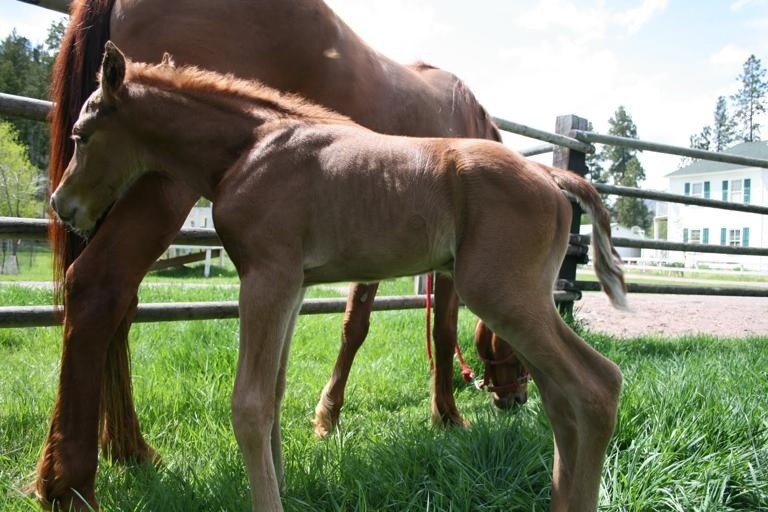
[47,39,638,512]
[34,0,532,511]
